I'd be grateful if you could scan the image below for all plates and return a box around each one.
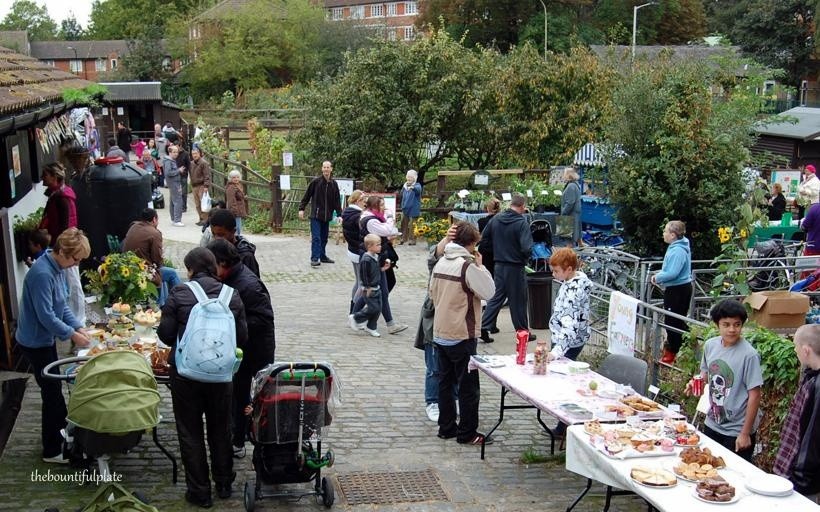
[548,364,569,376]
[621,397,663,415]
[584,428,595,436]
[105,323,136,332]
[745,472,794,497]
[693,492,739,505]
[630,479,676,488]
[663,461,700,484]
[112,310,133,318]
[672,439,703,449]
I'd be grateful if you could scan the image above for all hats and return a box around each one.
[805,165,816,173]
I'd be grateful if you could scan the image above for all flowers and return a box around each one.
[87,249,160,308]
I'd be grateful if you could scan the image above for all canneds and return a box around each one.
[693,375,704,396]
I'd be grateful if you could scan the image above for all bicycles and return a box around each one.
[570,226,629,293]
[784,239,815,277]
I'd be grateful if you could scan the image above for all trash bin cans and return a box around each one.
[523,272,554,330]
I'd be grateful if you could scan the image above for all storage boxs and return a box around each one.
[744,290,812,330]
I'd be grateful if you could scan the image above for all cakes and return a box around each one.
[585,395,699,455]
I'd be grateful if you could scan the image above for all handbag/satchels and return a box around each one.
[554,208,574,239]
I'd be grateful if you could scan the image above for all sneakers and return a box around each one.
[541,428,564,438]
[467,432,494,445]
[662,351,676,363]
[346,314,409,336]
[174,208,206,227]
[425,403,441,422]
[42,452,70,464]
[232,444,247,459]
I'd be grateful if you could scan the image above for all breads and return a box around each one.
[631,446,735,502]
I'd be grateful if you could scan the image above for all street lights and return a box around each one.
[631,2,661,75]
[67,46,79,77]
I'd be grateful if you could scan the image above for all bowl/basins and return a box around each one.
[564,362,590,381]
[627,416,640,425]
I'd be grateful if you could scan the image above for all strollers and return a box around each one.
[242,361,334,512]
[720,238,786,296]
[525,218,557,275]
[61,349,164,466]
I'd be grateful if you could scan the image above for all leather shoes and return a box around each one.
[215,480,233,498]
[529,332,536,341]
[489,327,499,333]
[185,489,214,508]
[479,329,493,343]
[311,260,321,266]
[321,256,334,263]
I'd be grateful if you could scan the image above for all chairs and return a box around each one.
[529,219,553,249]
[599,355,649,397]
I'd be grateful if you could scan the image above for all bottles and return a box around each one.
[229,348,244,372]
[533,339,548,376]
[514,329,529,365]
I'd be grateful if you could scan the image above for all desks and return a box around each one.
[563,417,820,512]
[750,217,808,244]
[468,353,687,512]
[449,210,558,234]
[62,309,181,487]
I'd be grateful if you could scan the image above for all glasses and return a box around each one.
[71,255,82,264]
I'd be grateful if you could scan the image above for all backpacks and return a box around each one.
[174,280,241,384]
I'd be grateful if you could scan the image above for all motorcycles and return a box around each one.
[151,170,165,209]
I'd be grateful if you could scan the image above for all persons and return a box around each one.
[731,163,820,292]
[398,167,421,245]
[17,105,399,470]
[419,192,535,445]
[560,168,583,248]
[683,299,819,498]
[542,245,589,436]
[651,220,695,366]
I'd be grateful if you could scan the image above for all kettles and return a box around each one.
[471,199,480,213]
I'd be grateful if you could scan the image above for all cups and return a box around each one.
[136,314,161,327]
[631,439,653,450]
[603,441,621,457]
[140,335,157,347]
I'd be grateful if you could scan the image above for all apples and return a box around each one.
[589,380,598,389]
